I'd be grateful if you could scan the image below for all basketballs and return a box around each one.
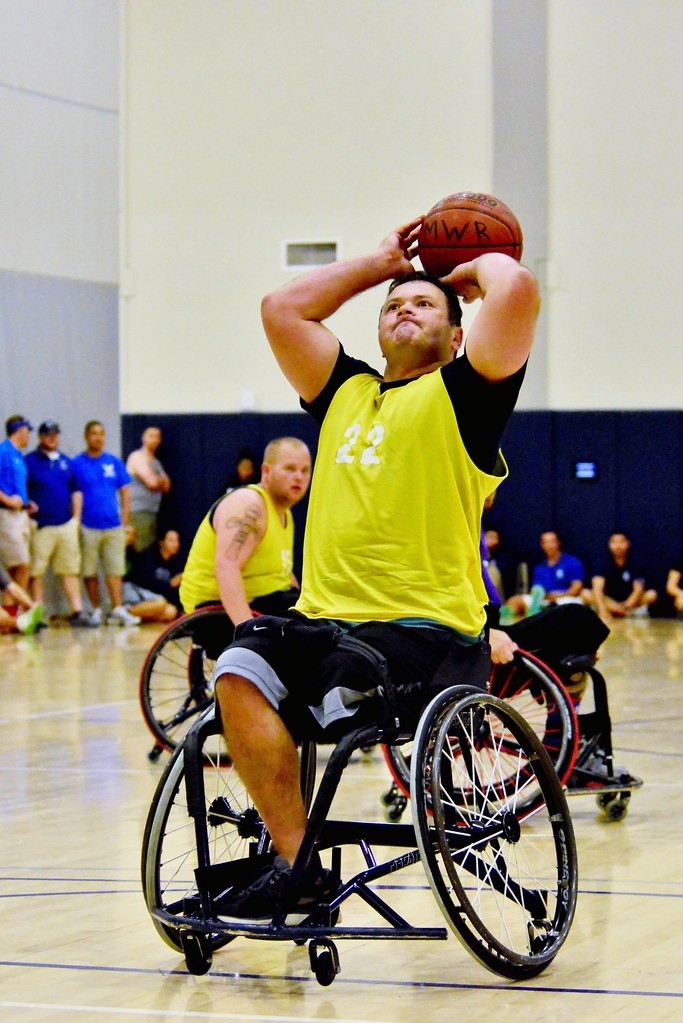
[418,191,524,293]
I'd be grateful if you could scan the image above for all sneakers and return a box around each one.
[71,615,101,627]
[216,865,342,926]
[92,607,102,623]
[566,738,628,788]
[107,606,142,627]
[15,602,44,636]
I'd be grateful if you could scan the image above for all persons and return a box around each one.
[479,487,643,792]
[209,212,542,936]
[177,437,312,662]
[221,452,261,494]
[485,529,683,624]
[0,415,183,634]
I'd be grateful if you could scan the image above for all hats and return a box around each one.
[8,420,34,434]
[38,420,61,434]
